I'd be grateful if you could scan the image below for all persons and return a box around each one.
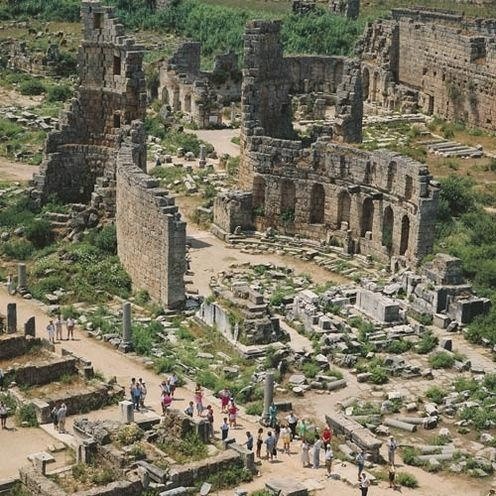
[360,473,370,496]
[129,378,147,412]
[46,314,75,343]
[356,450,365,481]
[386,462,397,490]
[156,150,161,167]
[158,373,334,478]
[49,401,68,434]
[386,435,397,466]
[0,401,8,429]
